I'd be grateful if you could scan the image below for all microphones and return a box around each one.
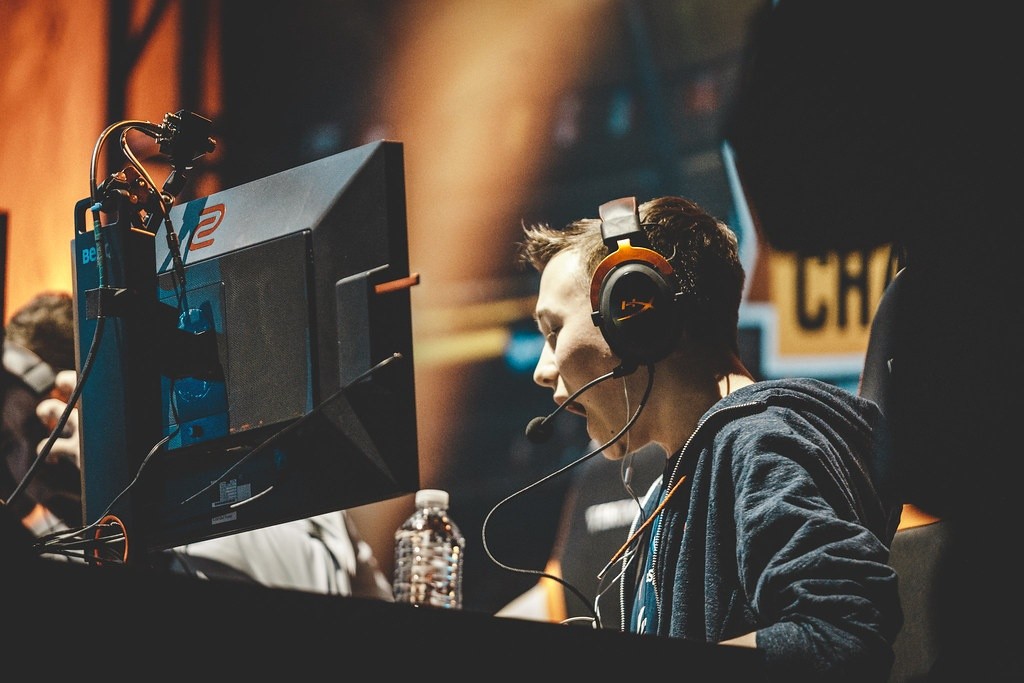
[526,358,640,444]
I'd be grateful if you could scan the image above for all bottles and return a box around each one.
[394,490,465,611]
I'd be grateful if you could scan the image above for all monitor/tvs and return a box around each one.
[69,137,422,564]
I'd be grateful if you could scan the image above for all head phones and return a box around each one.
[588,196,685,367]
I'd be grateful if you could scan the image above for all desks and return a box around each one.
[0,546,770,683]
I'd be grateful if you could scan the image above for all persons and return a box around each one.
[511,195,906,680]
[0,290,416,602]
